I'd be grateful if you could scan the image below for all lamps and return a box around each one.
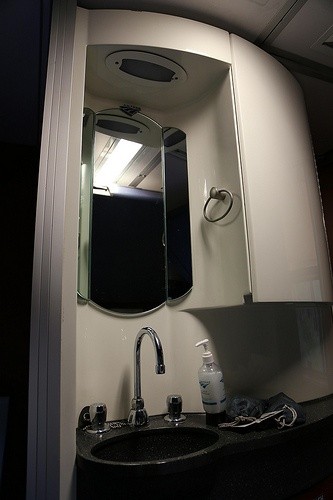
[103,50,187,89]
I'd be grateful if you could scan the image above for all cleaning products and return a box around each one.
[196,339,227,414]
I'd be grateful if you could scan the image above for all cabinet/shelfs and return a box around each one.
[226,31,333,305]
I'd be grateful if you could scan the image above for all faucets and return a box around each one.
[128,326,166,427]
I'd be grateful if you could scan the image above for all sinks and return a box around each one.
[74,416,227,500]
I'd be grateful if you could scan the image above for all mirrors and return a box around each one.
[77,106,193,319]
